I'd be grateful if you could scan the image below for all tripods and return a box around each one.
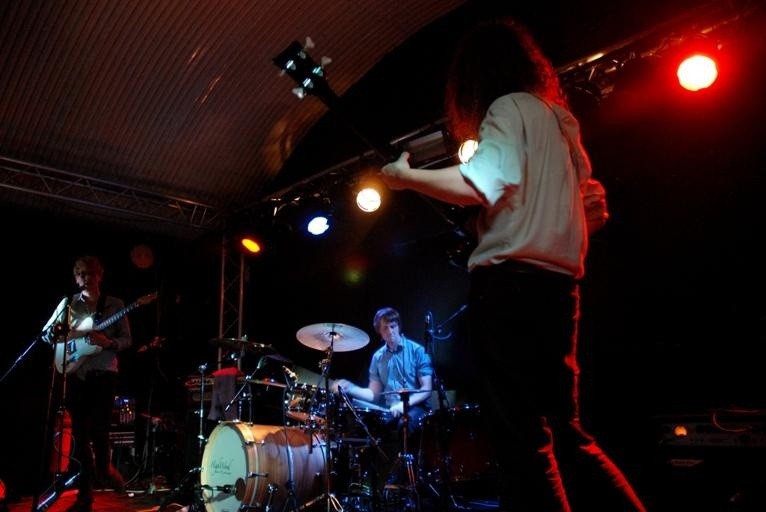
[292,404,441,512]
[157,366,208,512]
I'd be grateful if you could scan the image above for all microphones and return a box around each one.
[63,281,77,308]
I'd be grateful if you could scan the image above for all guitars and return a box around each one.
[55,293,158,373]
[276,37,479,270]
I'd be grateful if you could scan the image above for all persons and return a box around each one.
[327,303,435,440]
[378,16,648,511]
[39,254,133,494]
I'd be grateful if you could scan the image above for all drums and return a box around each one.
[342,440,397,497]
[337,407,392,438]
[422,406,480,482]
[201,420,333,512]
[284,383,339,425]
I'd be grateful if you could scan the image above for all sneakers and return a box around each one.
[65,499,93,512]
[113,478,126,494]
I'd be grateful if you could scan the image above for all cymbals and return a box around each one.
[212,338,274,354]
[296,323,370,352]
[379,389,428,394]
[246,379,287,388]
[250,343,291,362]
